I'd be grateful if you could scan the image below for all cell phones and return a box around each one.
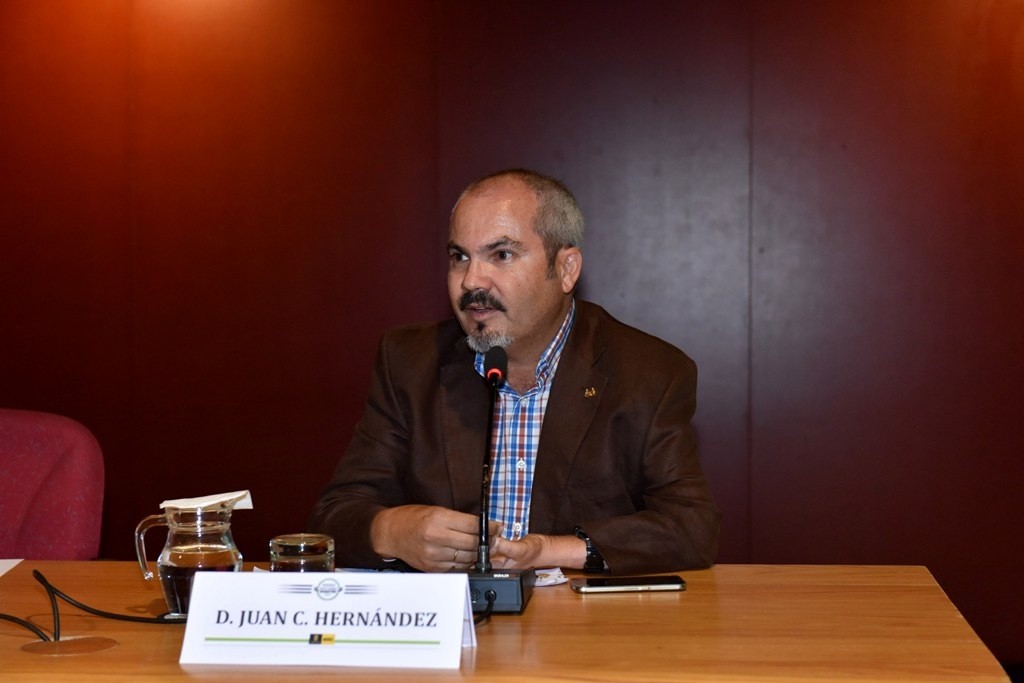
[572,575,687,593]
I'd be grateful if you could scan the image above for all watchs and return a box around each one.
[574,526,606,573]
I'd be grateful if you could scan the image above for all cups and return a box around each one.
[269,533,336,572]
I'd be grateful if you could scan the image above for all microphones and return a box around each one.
[437,346,537,615]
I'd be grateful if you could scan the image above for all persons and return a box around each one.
[307,168,720,576]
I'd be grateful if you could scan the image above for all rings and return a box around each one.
[452,548,459,562]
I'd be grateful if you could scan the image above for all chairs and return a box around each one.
[0,408,105,561]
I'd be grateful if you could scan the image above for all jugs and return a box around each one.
[135,499,243,619]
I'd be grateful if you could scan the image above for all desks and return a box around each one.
[0,559,1012,683]
